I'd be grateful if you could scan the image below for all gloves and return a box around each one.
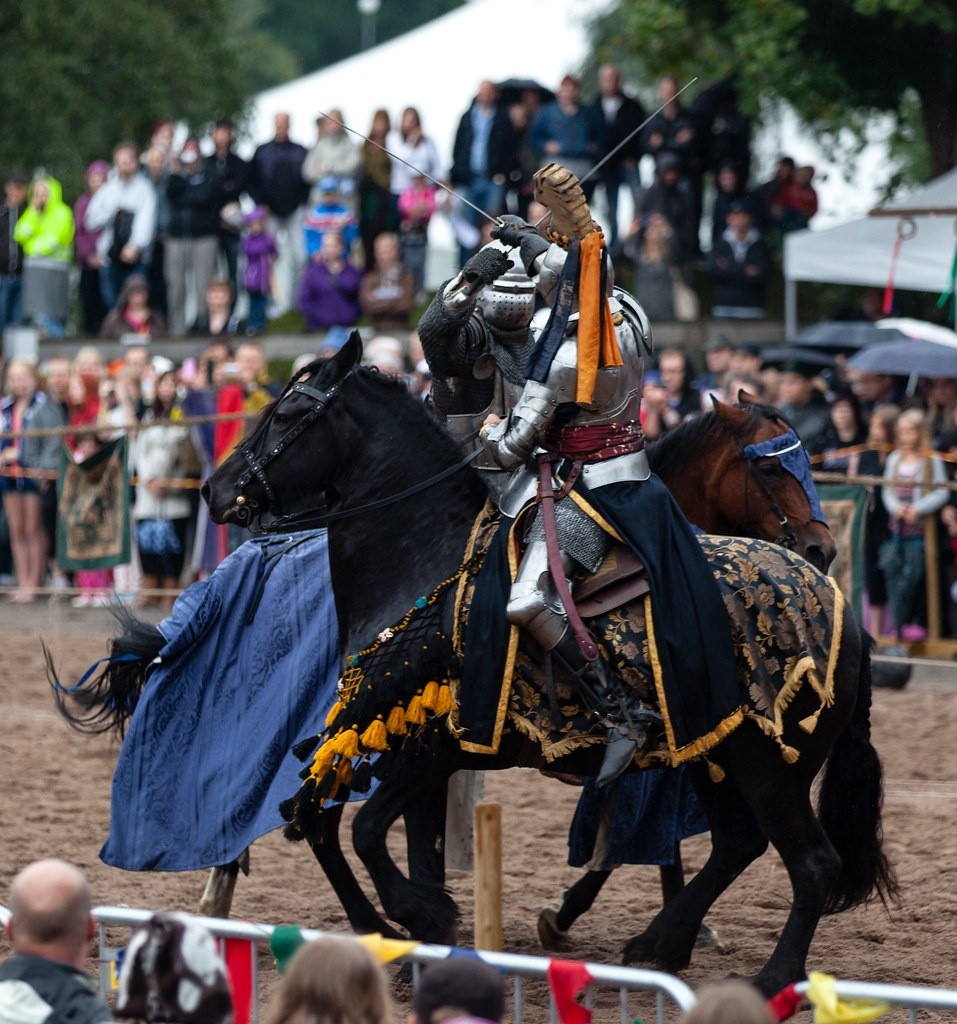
[490,214,551,278]
[461,246,514,286]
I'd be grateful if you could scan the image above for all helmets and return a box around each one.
[480,239,537,331]
[539,242,614,315]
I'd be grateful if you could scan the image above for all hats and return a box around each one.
[111,911,232,1022]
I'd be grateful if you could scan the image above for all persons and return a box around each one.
[5,61,957,1024]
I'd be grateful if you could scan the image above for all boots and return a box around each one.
[549,623,655,787]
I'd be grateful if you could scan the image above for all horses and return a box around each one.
[37,389,843,950]
[206,328,904,1014]
